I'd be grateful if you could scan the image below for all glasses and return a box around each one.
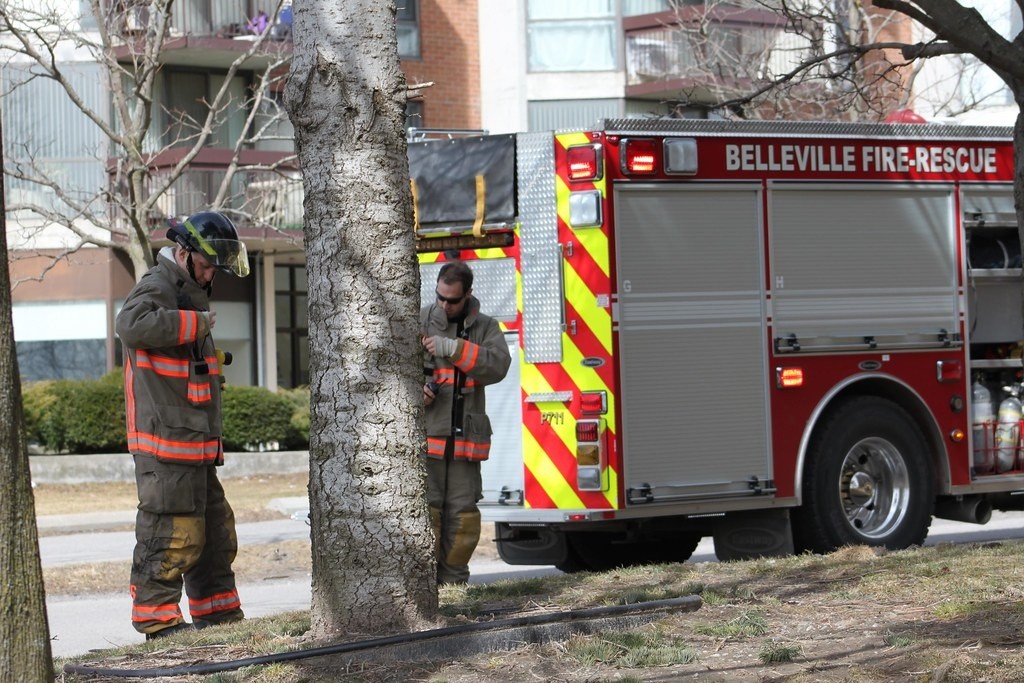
[434,285,465,305]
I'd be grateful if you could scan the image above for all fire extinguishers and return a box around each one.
[971,370,995,473]
[994,379,1023,473]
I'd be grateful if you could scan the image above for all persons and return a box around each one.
[114,210,250,640]
[418,260,511,584]
[216,11,269,39]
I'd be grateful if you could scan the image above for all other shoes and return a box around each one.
[146,622,192,640]
[195,618,246,630]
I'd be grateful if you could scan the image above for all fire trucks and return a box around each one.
[405,109,1023,573]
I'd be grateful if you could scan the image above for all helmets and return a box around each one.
[166,212,240,278]
